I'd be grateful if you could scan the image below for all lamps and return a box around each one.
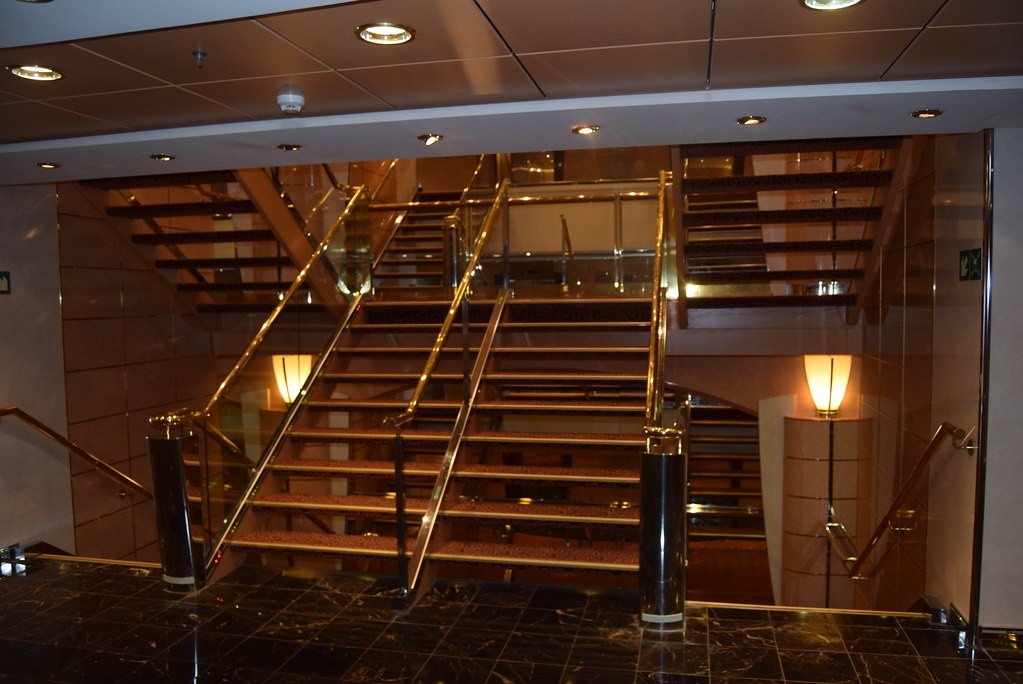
[271,354,313,408]
[802,354,853,415]
